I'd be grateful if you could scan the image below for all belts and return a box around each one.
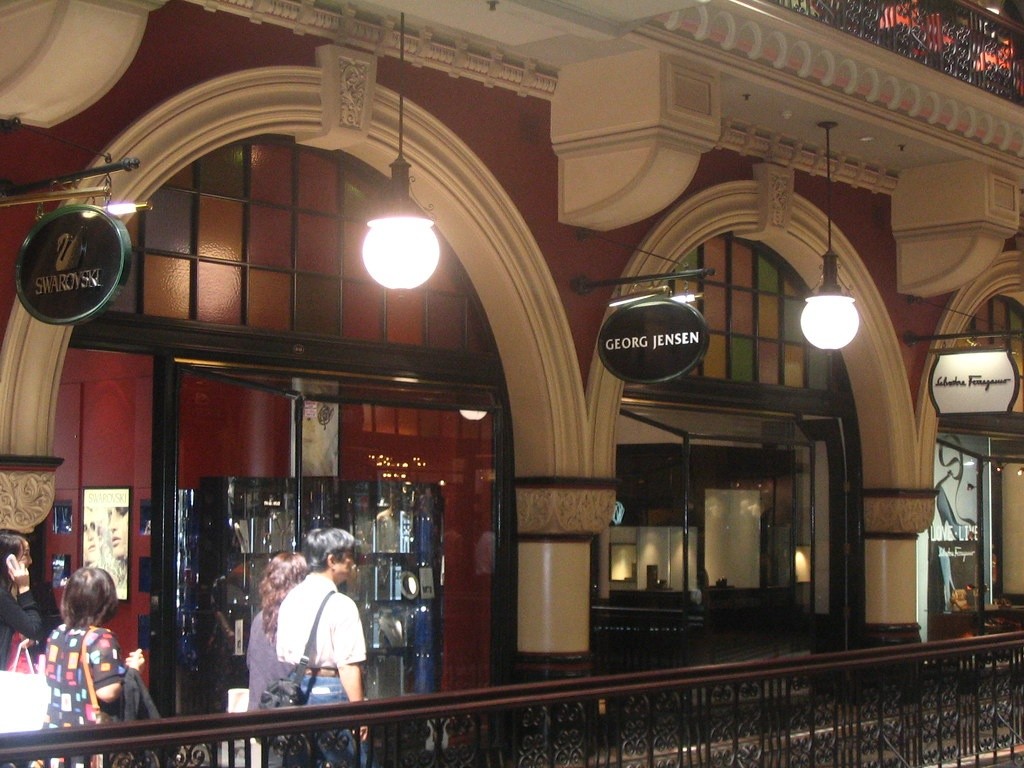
[304,667,340,676]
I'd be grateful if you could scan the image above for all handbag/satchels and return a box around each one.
[255,679,304,750]
[0,643,50,733]
[92,711,160,768]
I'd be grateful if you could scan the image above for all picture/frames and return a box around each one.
[79,486,133,602]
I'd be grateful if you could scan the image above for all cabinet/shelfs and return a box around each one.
[223,477,445,698]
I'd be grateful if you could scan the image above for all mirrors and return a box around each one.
[609,542,637,582]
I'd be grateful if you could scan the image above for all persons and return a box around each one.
[0,534,41,671]
[274,527,382,768]
[28,568,144,768]
[246,552,310,768]
[84,507,128,590]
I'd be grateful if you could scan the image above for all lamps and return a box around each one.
[102,202,154,216]
[362,12,440,290]
[670,293,703,304]
[801,122,860,349]
